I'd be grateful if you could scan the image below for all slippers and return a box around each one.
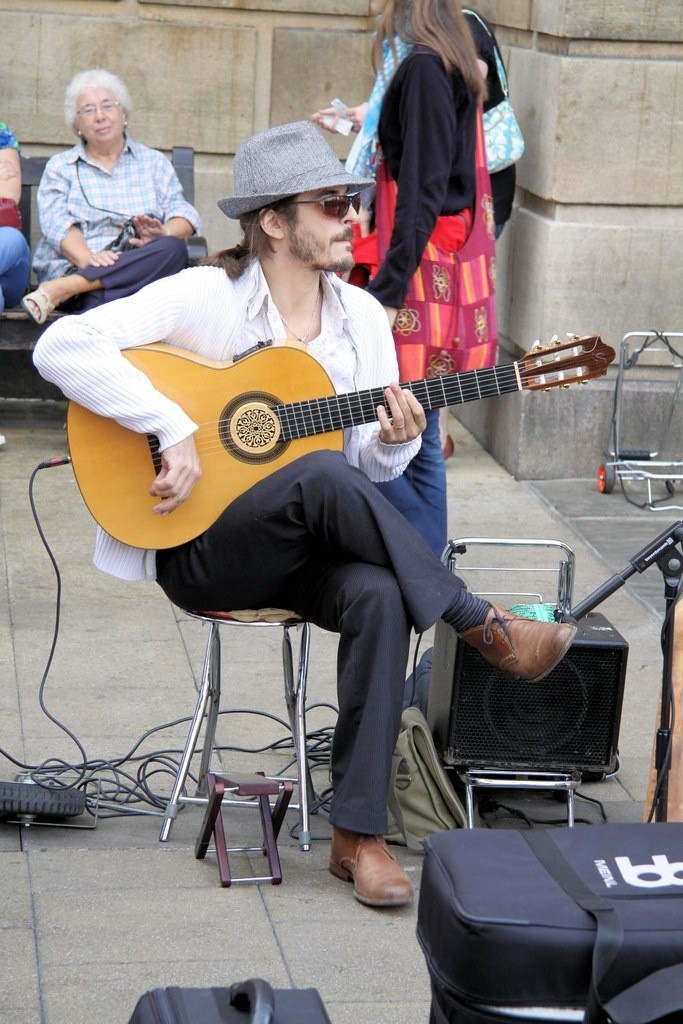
[20,282,57,326]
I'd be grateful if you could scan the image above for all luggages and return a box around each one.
[129,978,330,1024]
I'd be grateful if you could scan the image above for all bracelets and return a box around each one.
[161,224,172,237]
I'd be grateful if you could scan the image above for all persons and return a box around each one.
[309,0,516,567]
[22,67,199,324]
[0,120,32,446]
[28,121,578,906]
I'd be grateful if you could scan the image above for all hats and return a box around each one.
[216,121,376,219]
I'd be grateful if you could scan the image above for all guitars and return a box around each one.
[61,331,617,550]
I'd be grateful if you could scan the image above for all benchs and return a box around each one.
[0,146,209,351]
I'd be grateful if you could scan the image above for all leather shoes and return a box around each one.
[457,598,578,683]
[329,827,413,906]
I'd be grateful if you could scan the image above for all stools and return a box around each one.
[159,607,319,851]
[194,772,293,887]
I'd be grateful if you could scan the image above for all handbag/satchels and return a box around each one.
[375,229,499,383]
[0,198,22,229]
[381,706,467,853]
[415,821,683,1024]
[461,8,525,174]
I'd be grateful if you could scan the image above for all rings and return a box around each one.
[392,423,406,429]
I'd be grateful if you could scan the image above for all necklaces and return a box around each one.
[276,282,321,343]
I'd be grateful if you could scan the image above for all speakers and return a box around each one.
[427,610,629,774]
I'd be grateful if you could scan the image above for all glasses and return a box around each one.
[75,98,120,116]
[292,190,362,219]
